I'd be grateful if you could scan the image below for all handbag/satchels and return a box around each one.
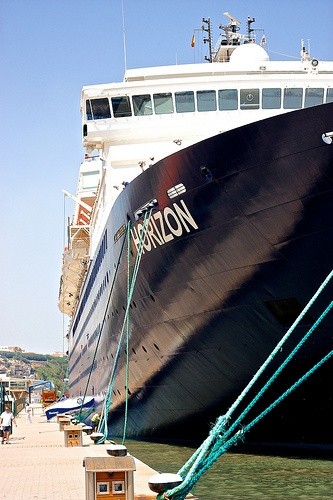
[0,430,3,437]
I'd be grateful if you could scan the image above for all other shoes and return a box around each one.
[6,442,11,444]
[2,441,4,443]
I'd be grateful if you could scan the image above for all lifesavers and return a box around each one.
[311,58,319,66]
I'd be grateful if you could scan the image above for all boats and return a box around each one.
[44,395,94,421]
[58,0,333,456]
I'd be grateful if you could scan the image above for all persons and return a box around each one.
[56,396,60,402]
[25,404,32,423]
[90,412,101,432]
[122,180,129,187]
[60,394,65,401]
[0,406,17,444]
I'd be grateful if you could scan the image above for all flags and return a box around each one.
[191,35,195,47]
